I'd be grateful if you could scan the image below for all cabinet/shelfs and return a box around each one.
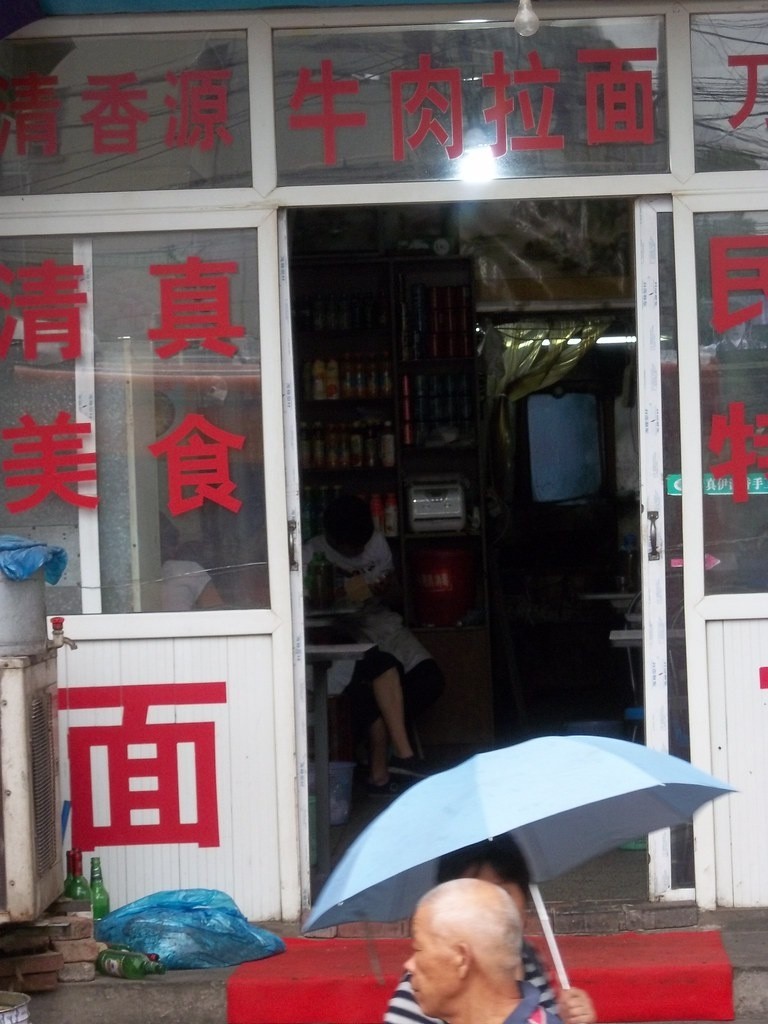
[286,248,502,747]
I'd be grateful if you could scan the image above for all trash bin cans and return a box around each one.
[308,762,357,826]
[566,720,624,740]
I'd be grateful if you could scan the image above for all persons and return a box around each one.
[382,830,593,1024]
[157,519,224,612]
[403,879,569,1024]
[299,492,436,803]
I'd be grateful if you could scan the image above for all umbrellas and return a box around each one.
[300,735,736,991]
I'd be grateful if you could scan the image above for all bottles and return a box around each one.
[60,847,165,980]
[284,268,488,557]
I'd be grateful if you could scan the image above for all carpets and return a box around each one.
[224,929,737,1022]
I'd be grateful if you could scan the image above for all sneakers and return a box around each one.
[389,755,442,778]
[369,780,407,796]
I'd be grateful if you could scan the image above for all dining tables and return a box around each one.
[304,604,426,876]
[577,565,690,741]
